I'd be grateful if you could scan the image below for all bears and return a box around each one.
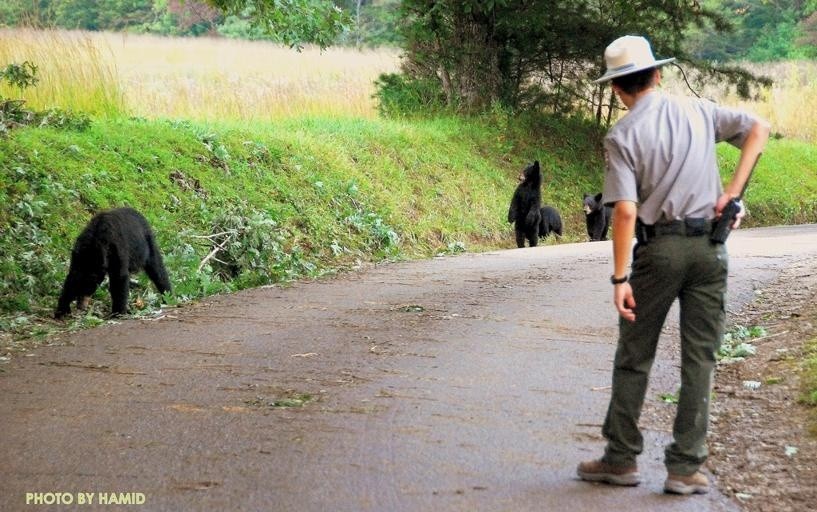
[54,207,172,320]
[538,206,562,238]
[507,159,543,249]
[582,192,611,242]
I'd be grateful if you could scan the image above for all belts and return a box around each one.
[638,216,714,237]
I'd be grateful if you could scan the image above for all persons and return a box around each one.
[575,35,770,495]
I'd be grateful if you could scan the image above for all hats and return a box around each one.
[592,34,675,84]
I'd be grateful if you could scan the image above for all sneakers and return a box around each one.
[662,470,711,495]
[575,457,641,487]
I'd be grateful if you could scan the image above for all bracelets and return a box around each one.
[610,273,627,286]
